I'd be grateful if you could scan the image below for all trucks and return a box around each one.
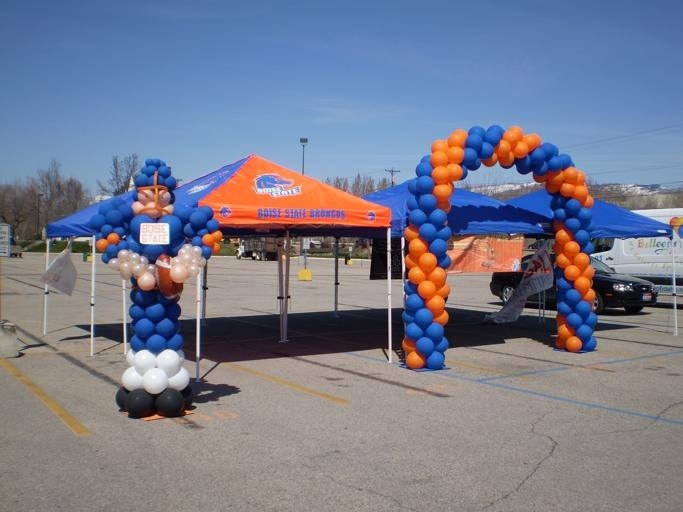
[236,237,279,260]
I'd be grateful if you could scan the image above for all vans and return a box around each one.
[589,209,683,283]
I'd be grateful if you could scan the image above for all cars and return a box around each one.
[488,254,658,314]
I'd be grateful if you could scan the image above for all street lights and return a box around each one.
[300,136,308,255]
[37,193,44,234]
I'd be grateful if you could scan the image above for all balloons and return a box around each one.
[92,159,227,420]
[401,124,598,370]
